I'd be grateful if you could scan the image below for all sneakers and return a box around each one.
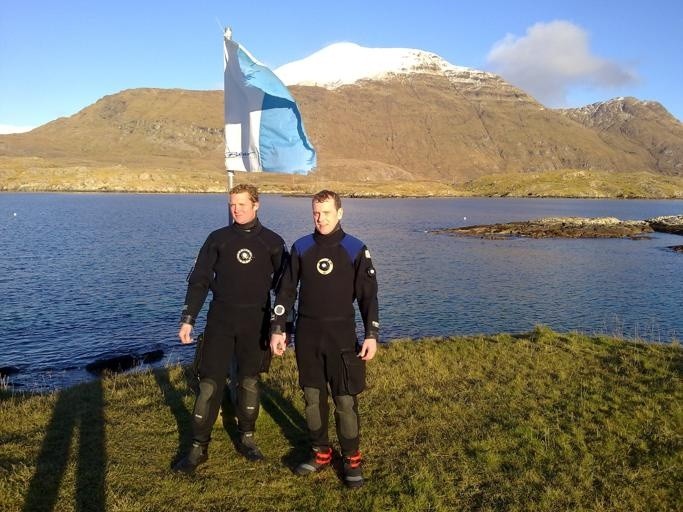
[342,450,364,487]
[171,442,208,473]
[294,448,332,476]
[236,431,263,461]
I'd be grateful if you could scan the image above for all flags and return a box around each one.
[224,34,317,175]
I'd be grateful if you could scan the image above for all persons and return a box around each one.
[270,190,379,488]
[173,184,297,476]
